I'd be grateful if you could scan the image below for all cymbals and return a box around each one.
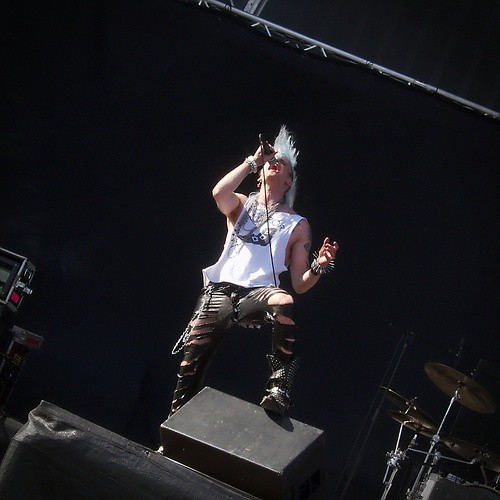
[424,359,499,416]
[386,410,436,440]
[442,435,500,474]
[379,385,436,425]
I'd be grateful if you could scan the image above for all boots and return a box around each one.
[260,353,301,414]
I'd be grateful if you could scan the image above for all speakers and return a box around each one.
[159,386,335,500]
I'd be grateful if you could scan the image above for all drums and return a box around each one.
[446,472,471,485]
[458,482,500,500]
[414,472,447,500]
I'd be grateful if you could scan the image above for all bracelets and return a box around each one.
[243,156,257,174]
[310,250,336,275]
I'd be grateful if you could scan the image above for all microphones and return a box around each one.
[259,134,273,155]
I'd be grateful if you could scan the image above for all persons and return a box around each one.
[154,123,339,464]
